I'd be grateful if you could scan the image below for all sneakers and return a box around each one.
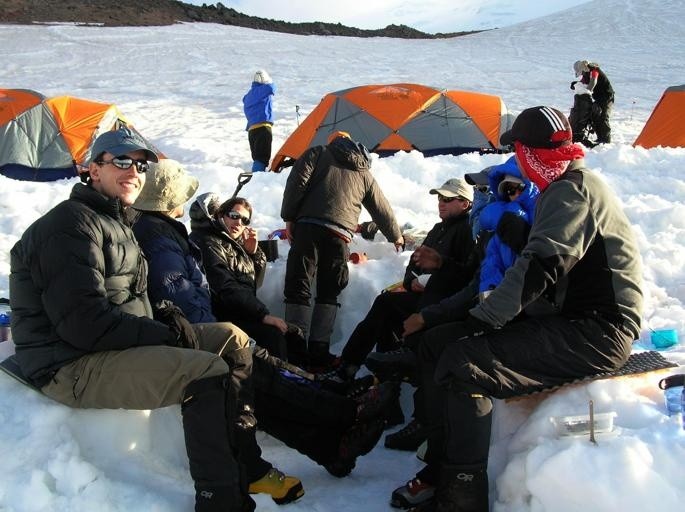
[249,468,305,504]
[391,476,439,509]
[306,352,429,477]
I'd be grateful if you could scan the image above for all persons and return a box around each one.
[339,107,645,512]
[574,60,615,144]
[9,129,386,512]
[280,131,404,355]
[243,70,277,174]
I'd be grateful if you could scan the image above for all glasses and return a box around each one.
[437,194,465,202]
[227,210,250,226]
[438,194,461,203]
[97,155,149,173]
[508,183,526,196]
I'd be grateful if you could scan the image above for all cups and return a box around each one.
[417,274,432,287]
[349,252,368,264]
[649,330,679,349]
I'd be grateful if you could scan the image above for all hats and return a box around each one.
[129,159,200,211]
[498,175,522,194]
[465,168,490,185]
[91,127,158,163]
[328,131,351,144]
[254,71,273,84]
[430,179,474,203]
[500,106,573,149]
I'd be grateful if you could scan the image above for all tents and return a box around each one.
[630,84,685,150]
[0,89,165,181]
[271,83,517,172]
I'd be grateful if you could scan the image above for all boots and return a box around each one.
[412,468,489,512]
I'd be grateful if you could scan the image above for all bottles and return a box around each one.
[659,374,684,429]
[0,313,12,343]
[271,231,282,240]
